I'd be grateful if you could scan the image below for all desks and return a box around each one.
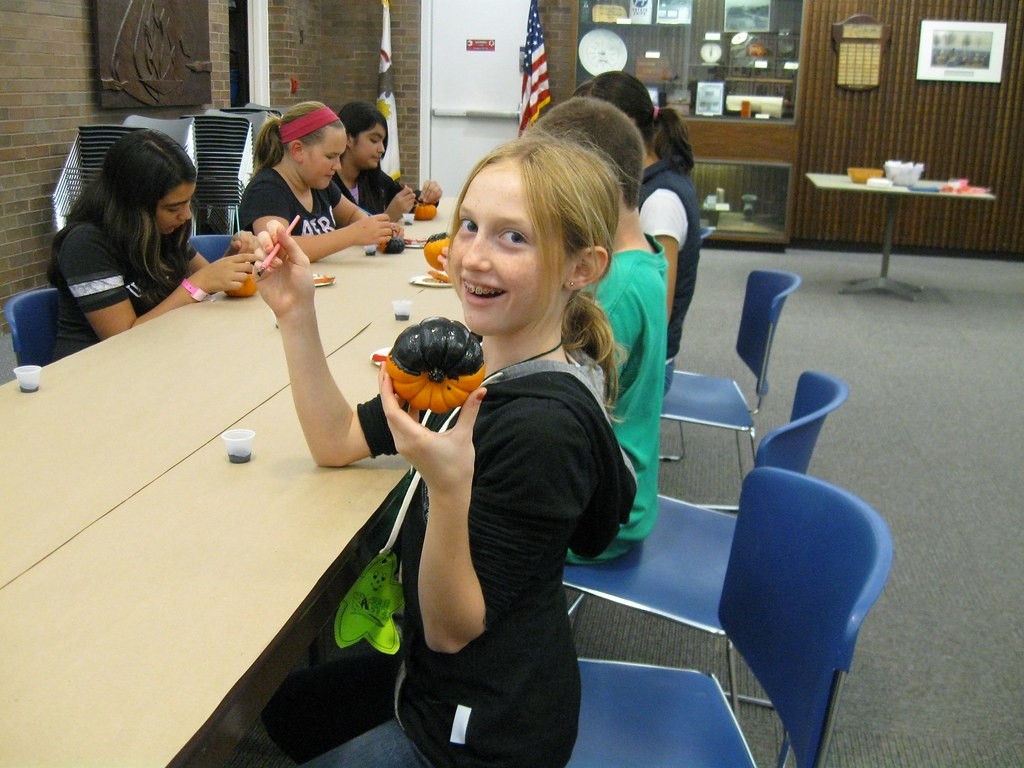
[802,171,996,302]
[1,207,503,768]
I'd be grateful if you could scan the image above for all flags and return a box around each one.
[375,0,401,182]
[516,0,550,136]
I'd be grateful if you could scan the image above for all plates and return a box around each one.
[409,274,452,287]
[312,273,335,287]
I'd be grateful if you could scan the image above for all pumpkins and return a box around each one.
[746,43,765,57]
[414,201,437,220]
[379,235,405,254]
[385,316,485,413]
[224,272,258,296]
[423,233,451,271]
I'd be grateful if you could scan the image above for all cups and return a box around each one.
[221,429,256,463]
[402,238,429,248]
[14,365,42,393]
[402,214,415,225]
[363,245,378,256]
[391,299,412,321]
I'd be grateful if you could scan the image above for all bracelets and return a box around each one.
[182,278,217,302]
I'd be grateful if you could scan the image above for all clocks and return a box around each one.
[579,27,631,81]
[695,41,723,66]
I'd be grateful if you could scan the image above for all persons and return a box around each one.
[573,71,701,398]
[331,102,443,222]
[44,128,261,360]
[528,96,668,566]
[252,127,637,768]
[234,101,404,264]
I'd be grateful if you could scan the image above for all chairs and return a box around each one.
[562,370,851,717]
[182,234,235,266]
[641,269,804,510]
[533,467,892,768]
[9,288,77,373]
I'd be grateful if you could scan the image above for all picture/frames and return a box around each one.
[717,0,774,34]
[918,20,1007,83]
[695,79,727,117]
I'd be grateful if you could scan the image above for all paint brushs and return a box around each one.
[260,214,301,273]
[234,204,241,236]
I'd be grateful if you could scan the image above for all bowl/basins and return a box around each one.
[847,167,883,185]
[885,164,924,187]
